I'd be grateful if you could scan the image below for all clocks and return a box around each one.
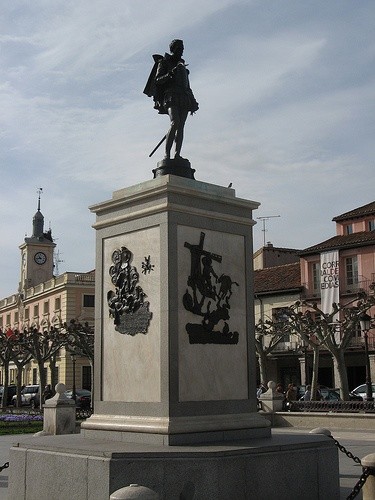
[34,251,47,265]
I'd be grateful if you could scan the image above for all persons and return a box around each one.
[43,384,54,404]
[257,381,322,411]
[153,39,200,161]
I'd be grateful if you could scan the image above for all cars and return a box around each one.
[65,390,91,408]
[283,385,340,402]
[348,383,375,407]
[0,384,16,406]
[12,384,50,408]
